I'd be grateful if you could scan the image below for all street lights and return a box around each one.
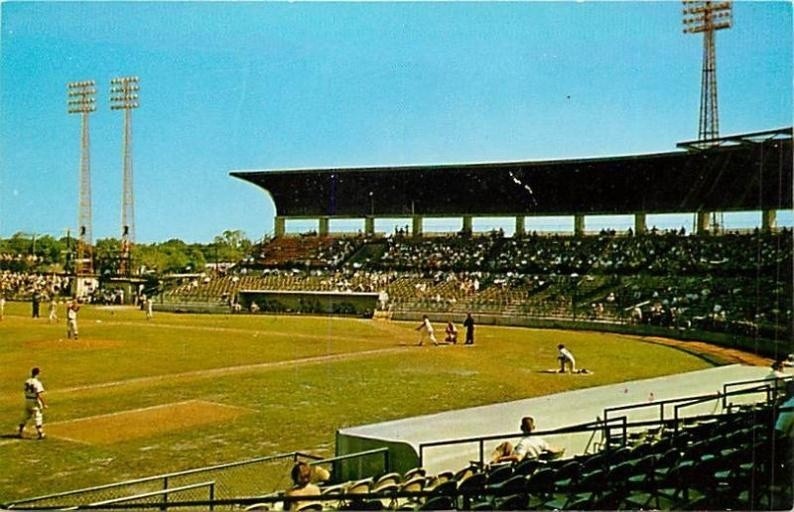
[104,77,142,277]
[64,80,97,275]
[677,4,732,146]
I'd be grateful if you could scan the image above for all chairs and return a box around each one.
[625,408,794,511]
[319,463,523,509]
[243,503,323,511]
[482,435,658,510]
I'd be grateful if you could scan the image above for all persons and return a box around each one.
[470,416,555,465]
[766,361,791,400]
[16,368,47,438]
[771,378,794,443]
[285,462,321,510]
[557,344,587,374]
